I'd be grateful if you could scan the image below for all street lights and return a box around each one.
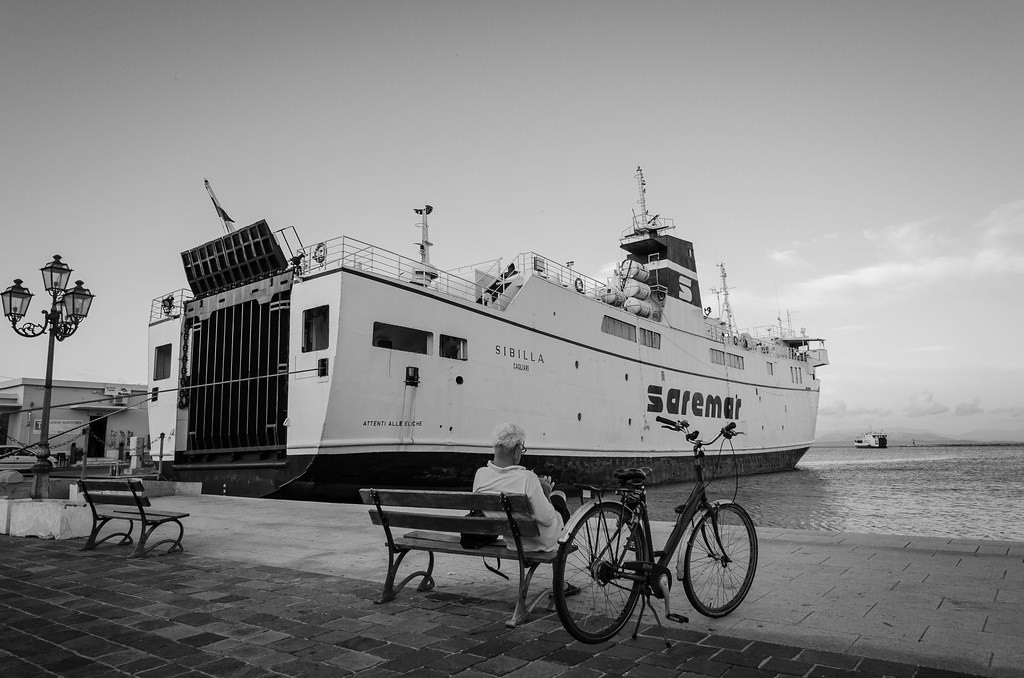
[1,255,96,499]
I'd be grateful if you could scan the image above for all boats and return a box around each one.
[855,430,888,450]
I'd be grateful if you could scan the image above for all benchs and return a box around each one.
[359,489,557,628]
[76,479,190,559]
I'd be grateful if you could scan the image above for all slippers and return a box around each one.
[549,582,577,598]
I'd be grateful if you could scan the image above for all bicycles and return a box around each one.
[560,419,758,644]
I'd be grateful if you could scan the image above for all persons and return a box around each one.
[472,424,581,599]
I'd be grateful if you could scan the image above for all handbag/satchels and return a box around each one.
[460,509,499,549]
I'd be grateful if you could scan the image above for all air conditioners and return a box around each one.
[114,396,128,404]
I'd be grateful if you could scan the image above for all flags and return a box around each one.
[205,186,235,223]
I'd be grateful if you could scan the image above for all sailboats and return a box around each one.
[913,438,915,445]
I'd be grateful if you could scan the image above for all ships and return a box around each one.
[148,167,825,491]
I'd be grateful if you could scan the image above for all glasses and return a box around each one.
[520,443,527,454]
